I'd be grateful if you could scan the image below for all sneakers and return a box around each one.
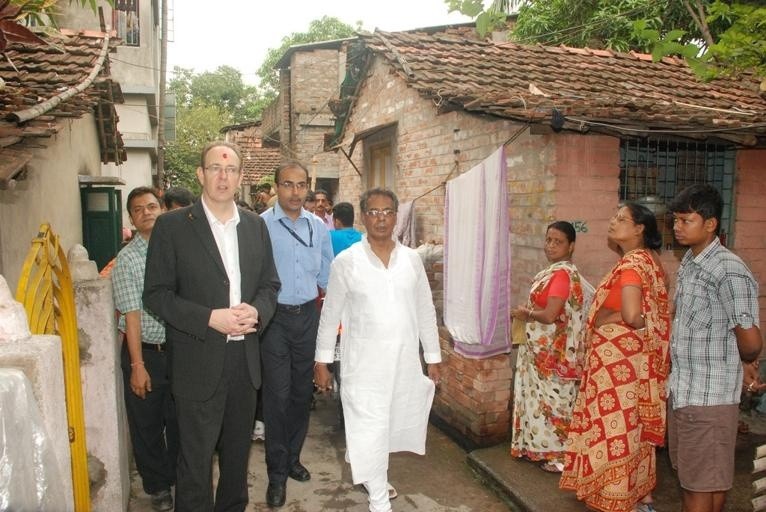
[252,420,266,441]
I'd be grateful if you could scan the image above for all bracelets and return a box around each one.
[129,361,143,368]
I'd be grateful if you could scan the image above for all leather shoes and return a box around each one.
[151,491,173,511]
[267,480,285,506]
[289,464,310,481]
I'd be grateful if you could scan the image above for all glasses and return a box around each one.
[281,181,310,189]
[367,209,396,216]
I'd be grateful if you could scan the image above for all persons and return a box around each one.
[259,161,335,509]
[303,189,363,258]
[511,220,586,475]
[115,184,173,510]
[558,203,671,511]
[159,186,192,211]
[313,187,444,512]
[665,188,763,511]
[141,140,281,512]
